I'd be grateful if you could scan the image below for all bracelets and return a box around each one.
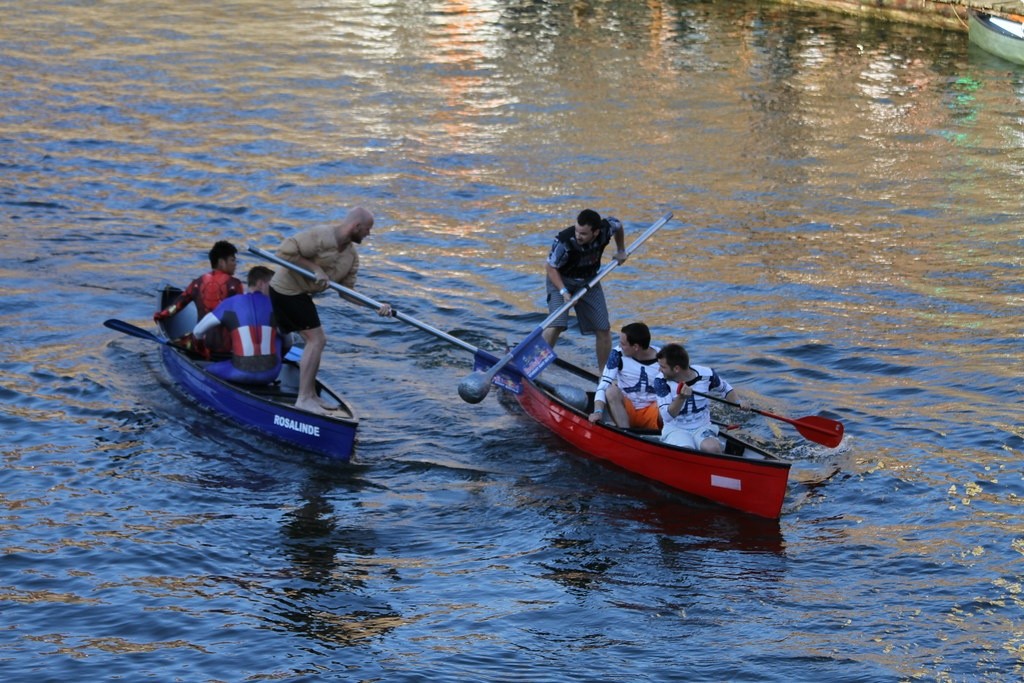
[618,250,625,253]
[594,410,603,413]
[735,400,742,403]
[559,288,567,295]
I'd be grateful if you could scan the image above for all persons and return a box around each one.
[589,323,660,432]
[543,209,627,374]
[193,266,282,384]
[154,241,245,361]
[654,344,752,455]
[268,207,393,415]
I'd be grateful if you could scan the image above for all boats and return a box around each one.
[506,332,792,520]
[966,7,1024,67]
[153,279,359,456]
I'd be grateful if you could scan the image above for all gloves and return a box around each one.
[152,309,170,324]
[189,334,207,354]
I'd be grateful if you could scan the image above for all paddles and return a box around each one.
[711,419,740,430]
[691,388,845,449]
[102,318,208,358]
